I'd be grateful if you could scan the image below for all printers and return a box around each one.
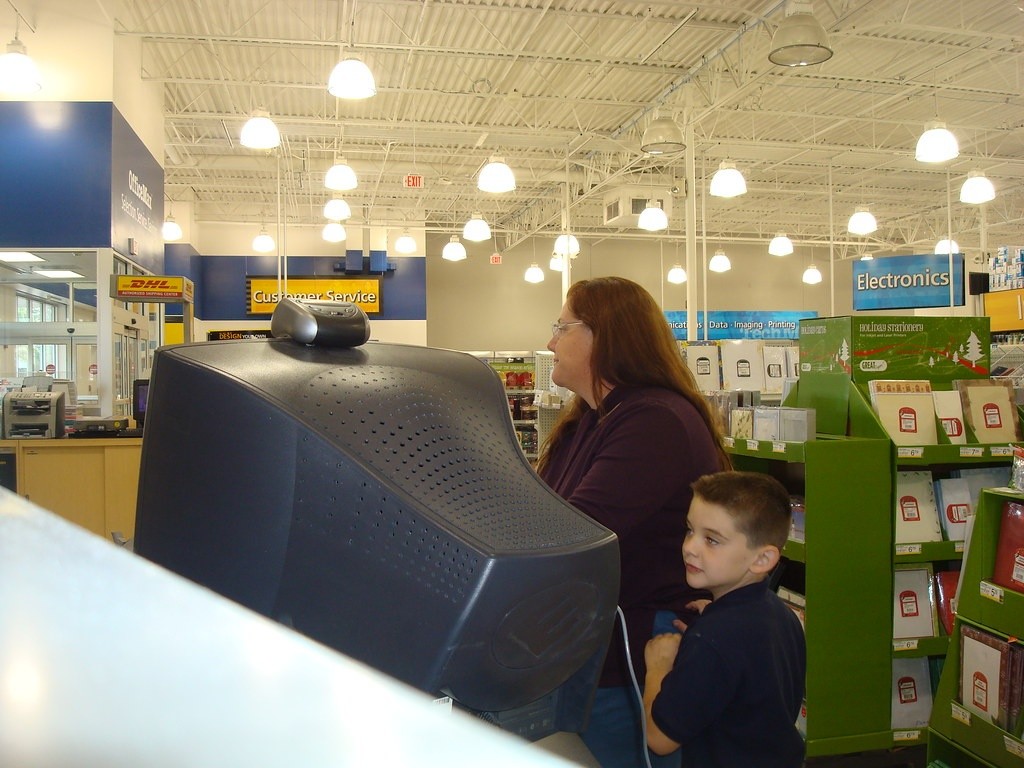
[3,391,65,439]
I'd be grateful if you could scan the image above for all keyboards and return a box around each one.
[118,428,144,438]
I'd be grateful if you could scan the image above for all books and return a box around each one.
[776,585,807,633]
[796,703,807,739]
[788,497,806,544]
[868,379,1024,732]
[686,339,816,442]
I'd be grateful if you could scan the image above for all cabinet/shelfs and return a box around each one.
[506,389,539,461]
[719,436,903,755]
[917,483,1022,767]
[875,414,1024,742]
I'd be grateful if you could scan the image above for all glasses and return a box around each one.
[551,320,582,336]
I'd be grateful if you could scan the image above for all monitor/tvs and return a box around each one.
[133,380,150,428]
[134,339,624,768]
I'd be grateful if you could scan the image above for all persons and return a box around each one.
[533,276,732,768]
[641,473,807,768]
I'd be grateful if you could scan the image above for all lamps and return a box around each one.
[327,1,375,99]
[477,141,516,196]
[441,207,466,261]
[164,200,183,241]
[253,204,274,253]
[392,225,417,254]
[768,1,834,68]
[463,206,490,242]
[636,91,999,285]
[0,10,53,101]
[523,226,584,284]
[320,222,347,243]
[322,194,350,221]
[323,124,360,193]
[237,71,278,148]
